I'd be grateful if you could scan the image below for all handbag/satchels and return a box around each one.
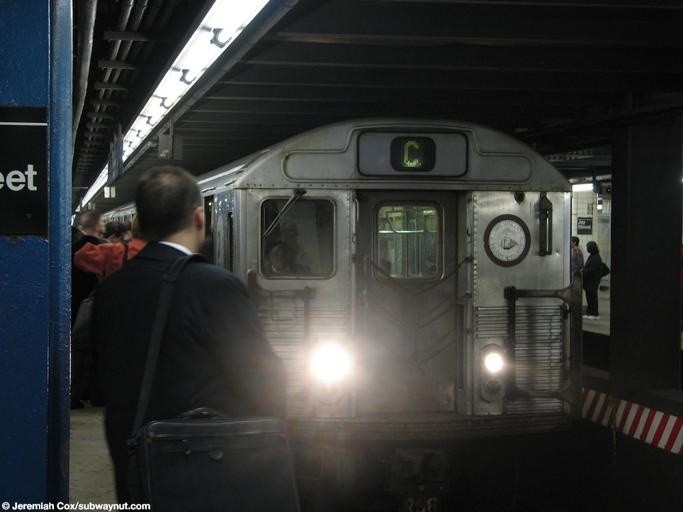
[601,263,610,277]
[127,404,301,512]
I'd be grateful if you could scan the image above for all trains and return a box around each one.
[101,119,582,511]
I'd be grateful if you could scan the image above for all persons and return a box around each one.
[88,166,288,512]
[580,240,603,320]
[571,237,584,283]
[266,236,310,275]
[70,208,148,409]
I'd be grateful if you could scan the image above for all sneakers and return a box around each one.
[582,315,600,320]
